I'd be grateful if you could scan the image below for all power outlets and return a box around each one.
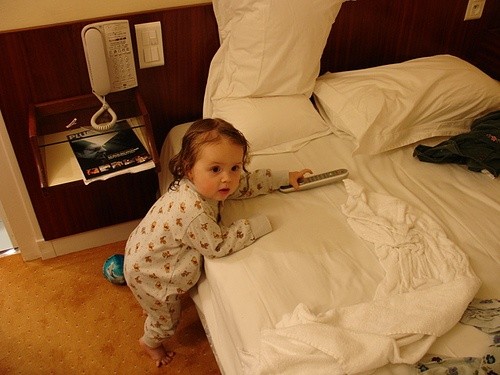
[463,0,486,21]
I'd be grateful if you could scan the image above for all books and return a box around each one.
[67,119,156,186]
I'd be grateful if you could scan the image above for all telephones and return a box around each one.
[80,20,139,131]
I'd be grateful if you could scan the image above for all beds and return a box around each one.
[158,98,500,375]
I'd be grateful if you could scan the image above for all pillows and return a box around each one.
[203,1,349,156]
[313,54,499,156]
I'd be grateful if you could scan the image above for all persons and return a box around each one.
[123,118,313,368]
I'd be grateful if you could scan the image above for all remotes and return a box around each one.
[278,169,349,193]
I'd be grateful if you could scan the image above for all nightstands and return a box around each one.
[28,89,161,241]
